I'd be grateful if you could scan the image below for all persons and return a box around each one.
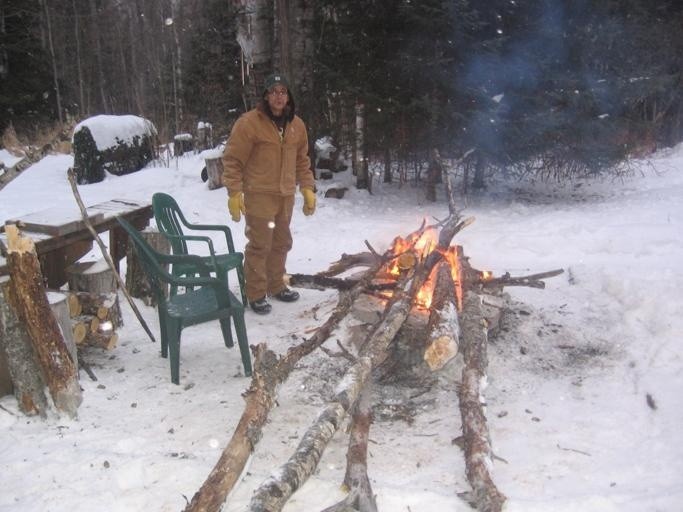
[423,147,443,202]
[220,73,318,314]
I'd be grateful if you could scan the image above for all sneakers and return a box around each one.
[270,288,299,303]
[248,299,272,316]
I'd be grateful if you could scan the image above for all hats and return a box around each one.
[261,71,295,124]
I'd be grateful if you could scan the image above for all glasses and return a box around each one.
[266,88,288,97]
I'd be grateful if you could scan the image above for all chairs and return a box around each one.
[115,214,252,385]
[150,191,249,308]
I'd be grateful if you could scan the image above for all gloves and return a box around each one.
[227,191,246,222]
[299,186,316,216]
